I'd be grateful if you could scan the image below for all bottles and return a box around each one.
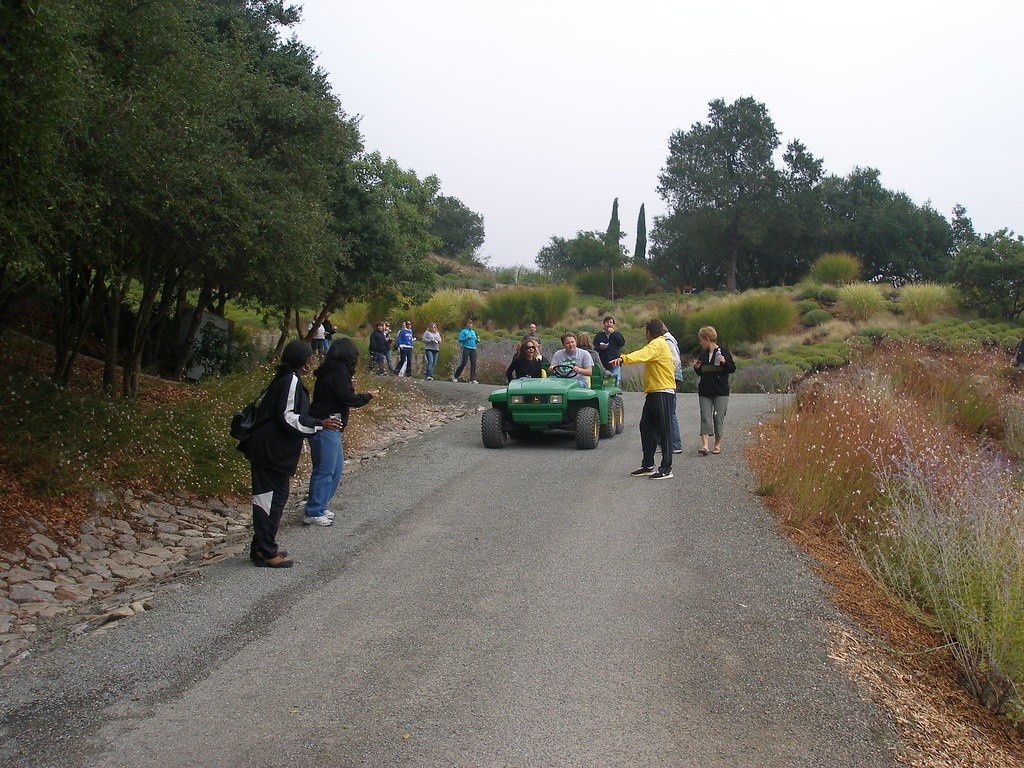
[477,335,480,339]
[715,348,721,365]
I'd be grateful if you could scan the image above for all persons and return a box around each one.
[422,323,442,381]
[369,320,417,378]
[521,321,540,343]
[609,318,676,479]
[547,331,594,388]
[307,311,337,358]
[301,338,379,526]
[593,316,625,387]
[451,319,481,384]
[236,339,343,568]
[577,332,613,377]
[656,320,683,453]
[692,326,736,455]
[506,340,549,389]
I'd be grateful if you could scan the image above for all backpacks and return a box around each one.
[229,402,265,443]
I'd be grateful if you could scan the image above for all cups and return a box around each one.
[329,413,341,419]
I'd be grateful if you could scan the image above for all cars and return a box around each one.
[481,362,625,449]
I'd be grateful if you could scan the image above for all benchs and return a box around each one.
[541,363,603,390]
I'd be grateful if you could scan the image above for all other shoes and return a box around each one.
[426,376,435,381]
[469,379,479,385]
[275,547,290,559]
[713,445,720,454]
[254,556,295,569]
[673,448,683,454]
[698,446,710,455]
[655,444,663,453]
[451,374,458,383]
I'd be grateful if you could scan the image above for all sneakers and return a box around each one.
[323,509,336,520]
[629,465,657,477]
[648,466,674,480]
[301,514,334,527]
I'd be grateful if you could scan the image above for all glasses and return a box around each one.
[386,324,391,327]
[406,323,412,325]
[526,346,536,349]
